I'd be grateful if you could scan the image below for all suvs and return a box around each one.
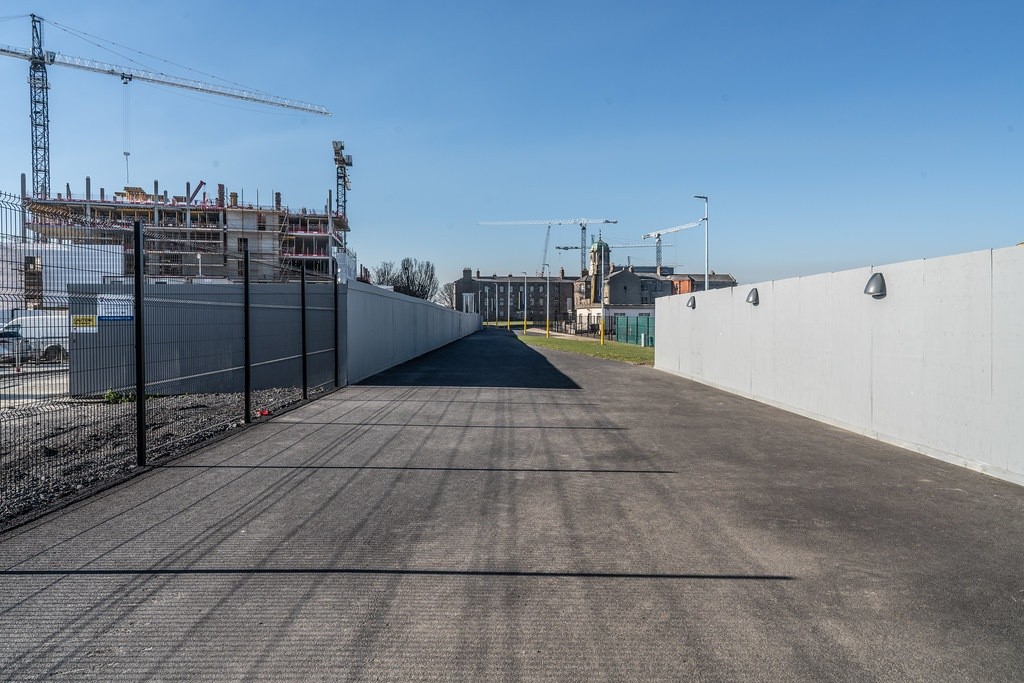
[0,330,35,365]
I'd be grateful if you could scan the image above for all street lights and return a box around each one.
[595,241,607,346]
[694,194,709,291]
[479,264,550,338]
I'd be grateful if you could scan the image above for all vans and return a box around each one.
[0,314,72,364]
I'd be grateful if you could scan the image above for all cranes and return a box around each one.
[330,137,356,255]
[556,234,671,253]
[640,221,700,268]
[474,215,618,273]
[0,11,330,204]
